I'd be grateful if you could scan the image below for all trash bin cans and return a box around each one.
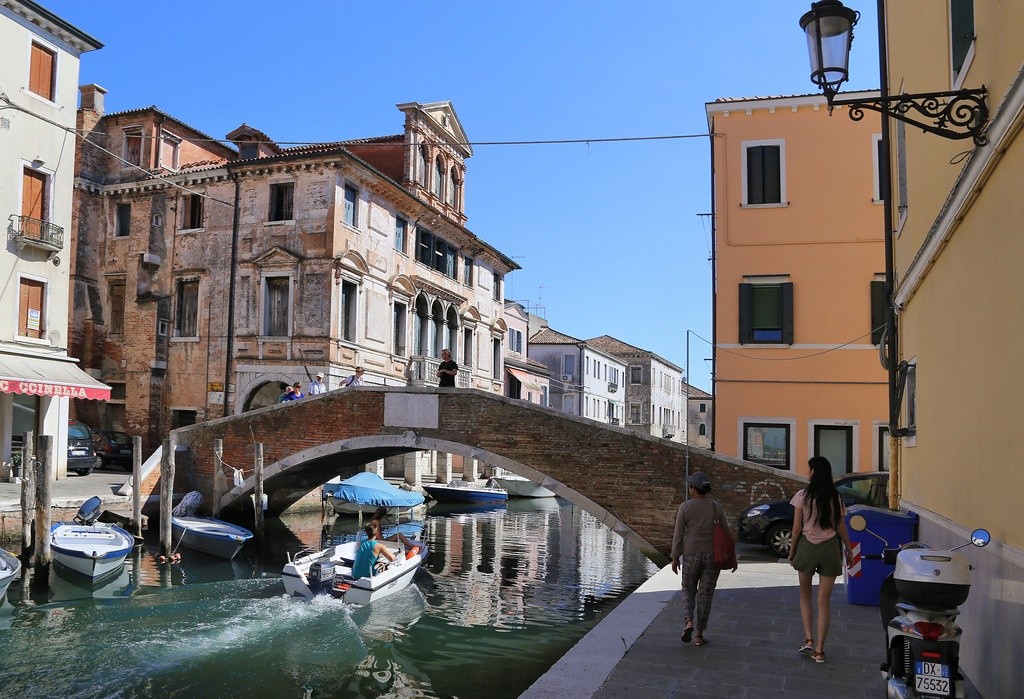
[842,503,919,607]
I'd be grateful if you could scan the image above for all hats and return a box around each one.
[316,372,324,378]
[684,471,709,491]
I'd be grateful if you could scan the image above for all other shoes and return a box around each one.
[694,636,709,646]
[681,620,693,643]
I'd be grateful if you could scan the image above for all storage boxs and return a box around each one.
[893,549,972,610]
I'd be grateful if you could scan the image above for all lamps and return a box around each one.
[799,0,990,148]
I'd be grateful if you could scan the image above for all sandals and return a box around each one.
[811,649,825,663]
[799,638,814,653]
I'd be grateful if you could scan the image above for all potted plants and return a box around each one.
[12,454,22,477]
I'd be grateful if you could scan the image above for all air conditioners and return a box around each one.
[560,374,572,382]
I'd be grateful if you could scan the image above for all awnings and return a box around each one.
[506,367,543,395]
[0,347,111,401]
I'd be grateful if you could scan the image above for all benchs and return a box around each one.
[334,564,354,577]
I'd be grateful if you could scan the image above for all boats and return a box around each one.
[171,515,254,561]
[48,496,136,585]
[406,500,509,522]
[491,475,560,497]
[321,470,432,513]
[282,540,430,607]
[0,549,22,601]
[421,479,508,501]
[36,558,140,609]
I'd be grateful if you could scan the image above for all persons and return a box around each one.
[670,471,738,646]
[787,456,853,662]
[307,372,326,396]
[340,366,364,388]
[284,382,305,402]
[437,349,459,388]
[278,386,293,403]
[351,506,424,582]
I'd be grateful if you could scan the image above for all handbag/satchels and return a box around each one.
[712,500,736,570]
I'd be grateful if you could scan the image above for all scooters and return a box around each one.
[849,513,991,699]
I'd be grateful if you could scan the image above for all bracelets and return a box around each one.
[788,553,793,556]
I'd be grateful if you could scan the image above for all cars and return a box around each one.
[738,470,888,558]
[91,428,133,473]
[67,419,98,476]
